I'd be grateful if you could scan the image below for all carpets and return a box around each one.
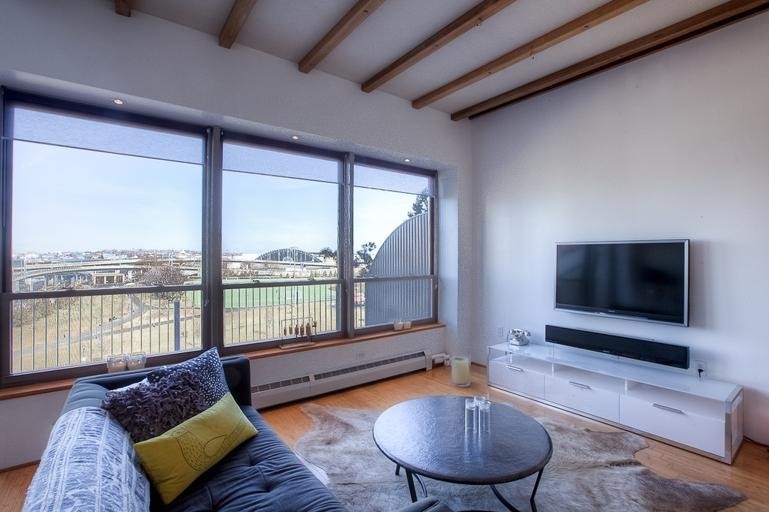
[294,401,750,512]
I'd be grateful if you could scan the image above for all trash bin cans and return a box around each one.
[450,356,470,384]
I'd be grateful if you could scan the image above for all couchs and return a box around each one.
[21,355,352,511]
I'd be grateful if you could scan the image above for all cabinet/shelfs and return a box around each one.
[487,339,745,466]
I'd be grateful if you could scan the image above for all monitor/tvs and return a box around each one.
[554,238,689,328]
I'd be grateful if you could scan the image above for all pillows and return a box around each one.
[99,345,260,506]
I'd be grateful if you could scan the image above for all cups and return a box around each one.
[473,395,492,436]
[464,398,478,436]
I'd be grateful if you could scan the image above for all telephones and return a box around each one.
[508,329,531,346]
